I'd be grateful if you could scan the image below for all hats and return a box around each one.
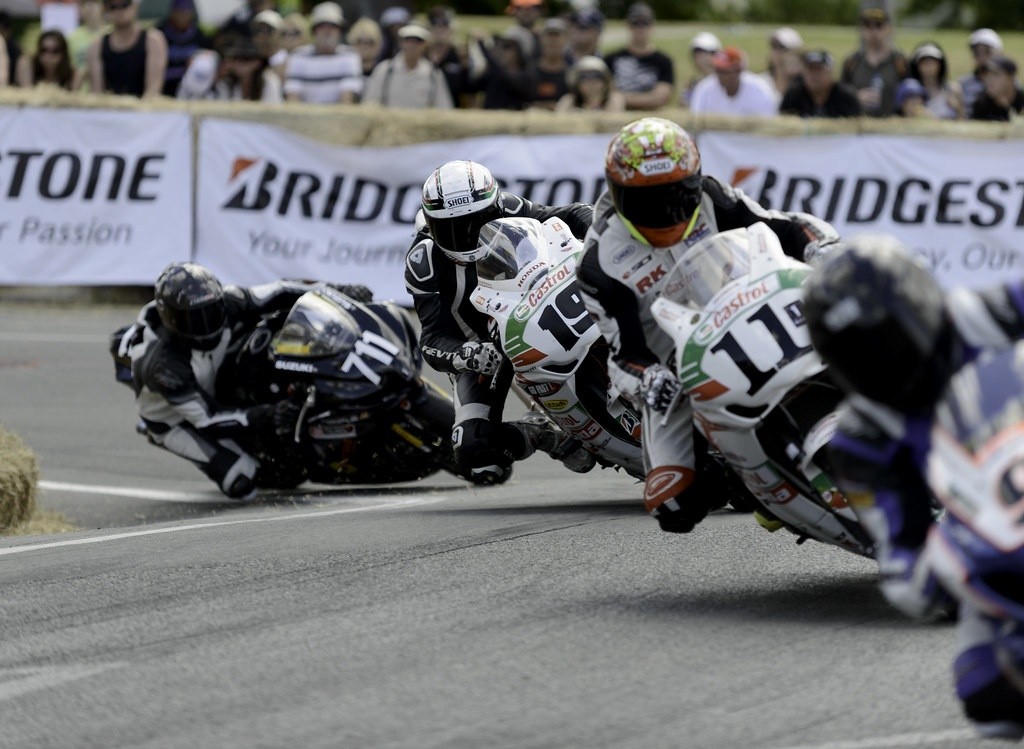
[971,27,1004,46]
[897,80,929,104]
[225,0,889,85]
[915,44,944,62]
[970,56,1016,76]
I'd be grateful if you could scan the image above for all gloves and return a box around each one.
[325,280,374,305]
[452,338,503,375]
[639,363,687,414]
[251,400,301,438]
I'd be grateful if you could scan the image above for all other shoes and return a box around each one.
[249,465,310,490]
[720,464,754,517]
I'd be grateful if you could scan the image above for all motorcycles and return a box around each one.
[457,215,758,515]
[636,224,881,558]
[896,336,1024,723]
[111,282,458,489]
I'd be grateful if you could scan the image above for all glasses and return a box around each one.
[104,2,131,11]
[38,46,61,55]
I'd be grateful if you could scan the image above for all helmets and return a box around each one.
[604,113,704,244]
[803,230,951,417]
[421,160,504,262]
[152,261,229,350]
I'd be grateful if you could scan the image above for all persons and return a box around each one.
[758,27,806,98]
[575,116,843,533]
[679,31,722,108]
[803,234,1024,731]
[0,0,675,112]
[959,28,1018,121]
[841,6,908,120]
[127,261,373,499]
[893,39,969,121]
[778,49,859,119]
[689,47,780,118]
[404,159,597,477]
[968,58,1024,121]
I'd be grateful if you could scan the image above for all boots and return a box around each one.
[503,410,595,473]
